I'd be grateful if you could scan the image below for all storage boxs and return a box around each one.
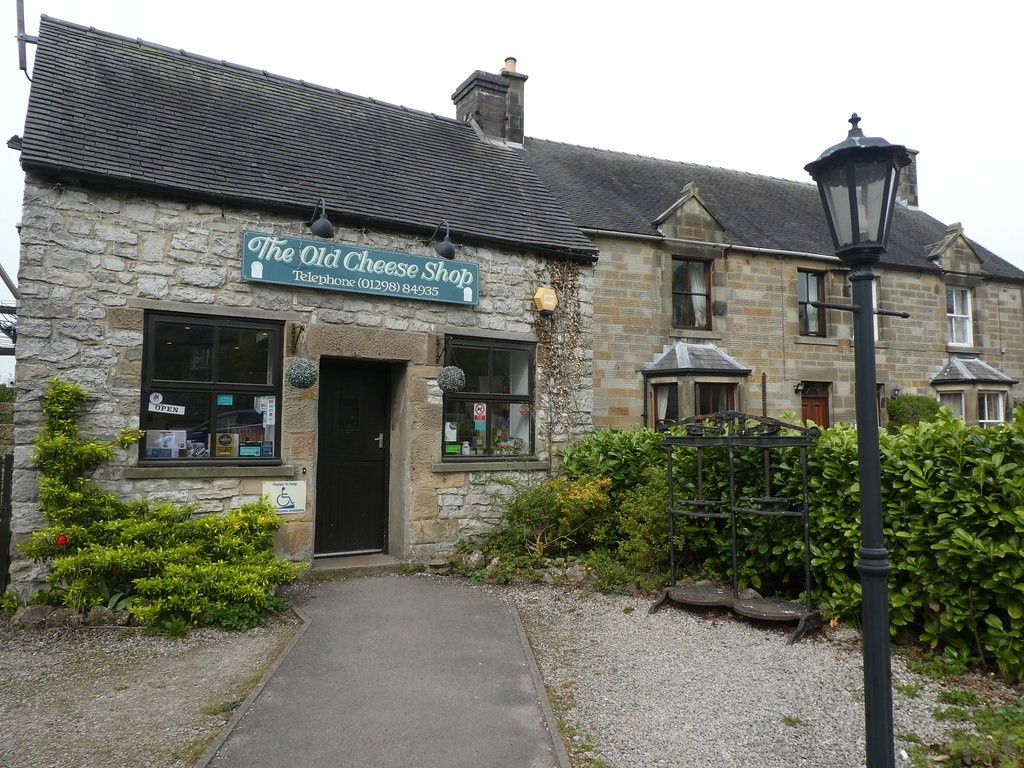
[445,445,461,453]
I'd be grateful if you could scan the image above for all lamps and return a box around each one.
[305,198,335,239]
[6,135,23,151]
[891,386,900,399]
[422,221,455,260]
[795,383,805,394]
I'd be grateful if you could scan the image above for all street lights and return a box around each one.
[803,110,913,768]
